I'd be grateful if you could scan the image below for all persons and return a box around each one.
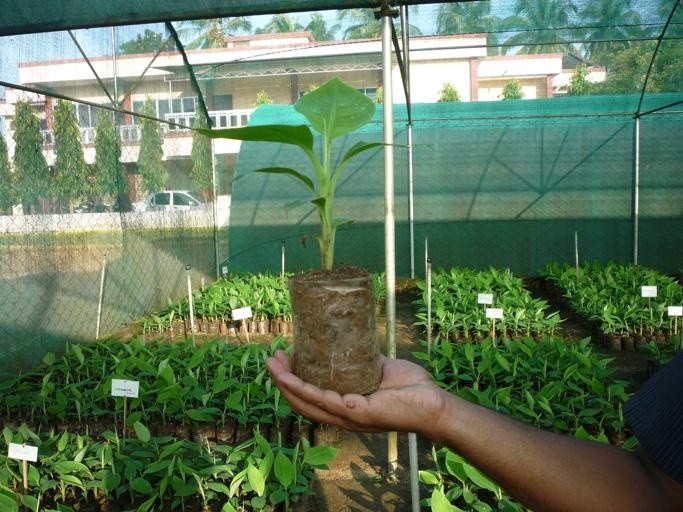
[266,350,683,511]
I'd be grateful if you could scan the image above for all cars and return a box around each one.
[141,188,211,210]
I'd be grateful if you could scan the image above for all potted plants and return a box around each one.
[0,268,387,512]
[189,77,409,394]
[411,259,683,511]
